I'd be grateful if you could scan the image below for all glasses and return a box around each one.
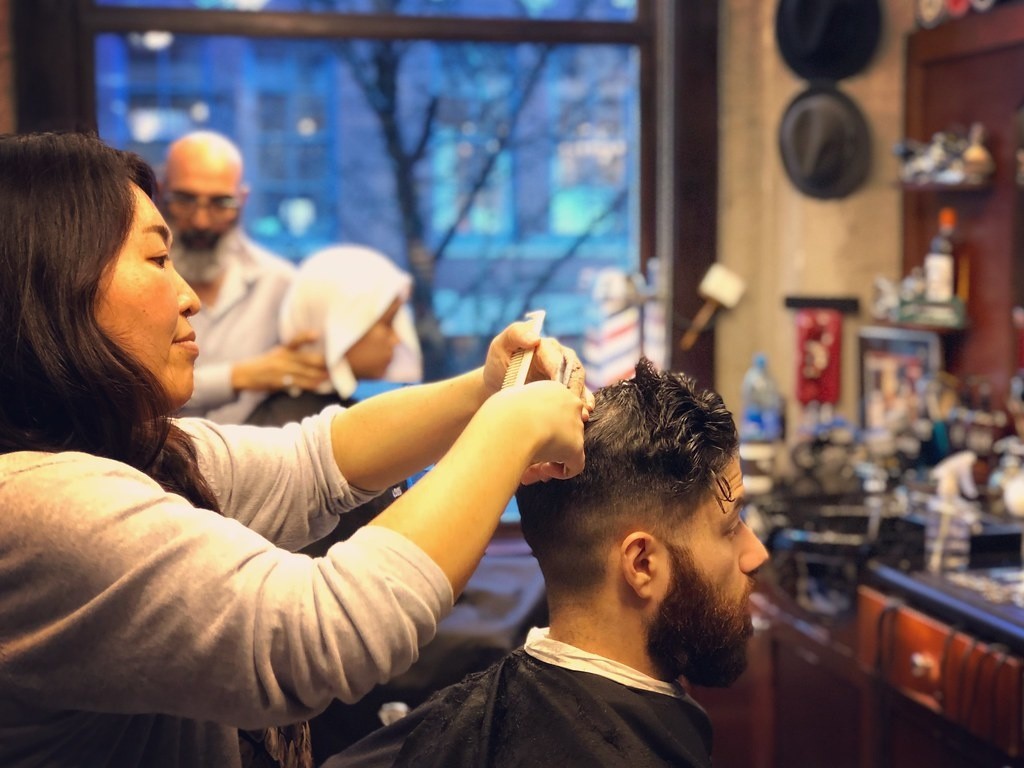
[158,184,244,210]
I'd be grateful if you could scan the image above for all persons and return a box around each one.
[0,133,595,768]
[125,133,330,427]
[444,360,773,766]
[272,240,411,552]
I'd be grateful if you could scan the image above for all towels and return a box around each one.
[273,239,426,402]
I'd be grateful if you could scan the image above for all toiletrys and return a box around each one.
[735,336,785,448]
[925,448,982,576]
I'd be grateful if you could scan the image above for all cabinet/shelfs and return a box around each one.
[743,592,872,768]
[857,585,1024,768]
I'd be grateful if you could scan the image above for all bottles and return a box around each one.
[741,354,779,445]
[918,211,960,304]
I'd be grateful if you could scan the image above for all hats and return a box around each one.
[777,86,871,198]
[774,0,882,85]
